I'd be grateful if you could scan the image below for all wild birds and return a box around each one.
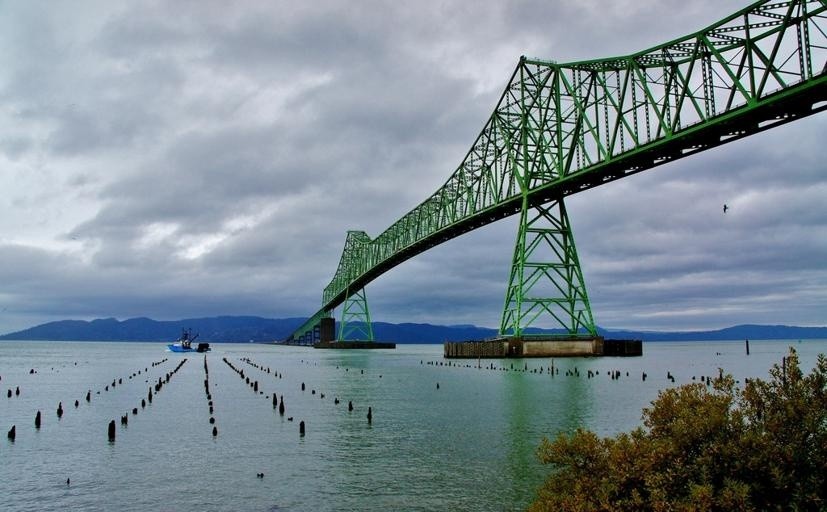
[723,204,727,213]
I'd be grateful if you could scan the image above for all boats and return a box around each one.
[167,327,211,353]
